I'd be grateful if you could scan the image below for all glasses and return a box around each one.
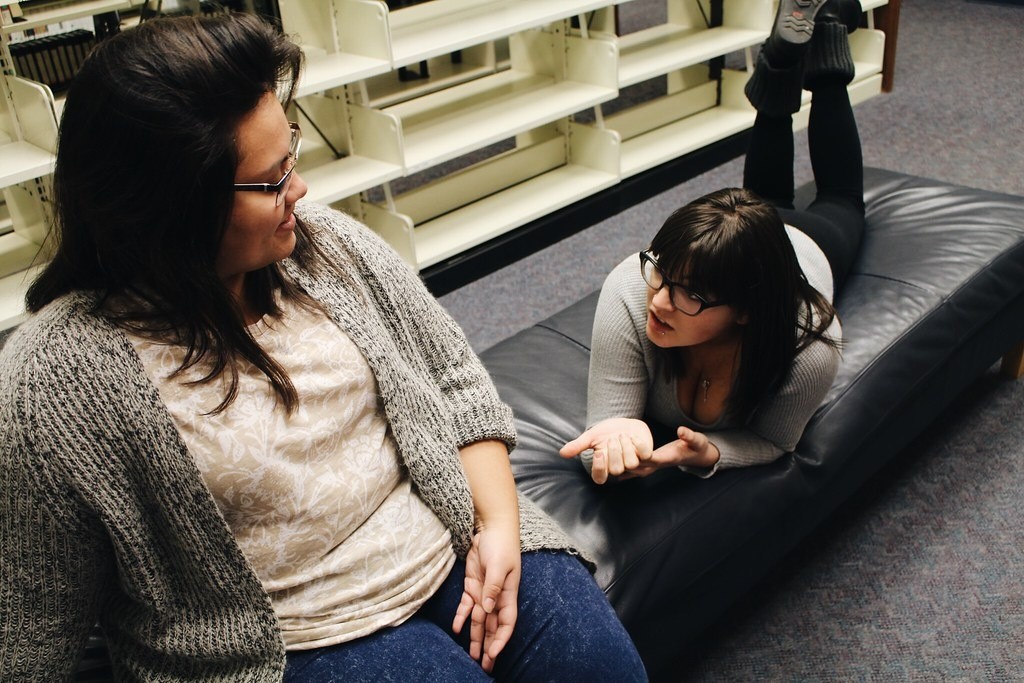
[639,247,727,316]
[231,120,303,207]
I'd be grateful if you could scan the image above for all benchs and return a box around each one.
[74,166,1024,683]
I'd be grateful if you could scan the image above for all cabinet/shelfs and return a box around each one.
[0,0,902,350]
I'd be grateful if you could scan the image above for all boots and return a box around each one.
[745,0,827,118]
[803,0,862,92]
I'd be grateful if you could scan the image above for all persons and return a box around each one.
[0,9,649,683]
[558,0,866,485]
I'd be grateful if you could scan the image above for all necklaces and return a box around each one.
[687,346,732,403]
